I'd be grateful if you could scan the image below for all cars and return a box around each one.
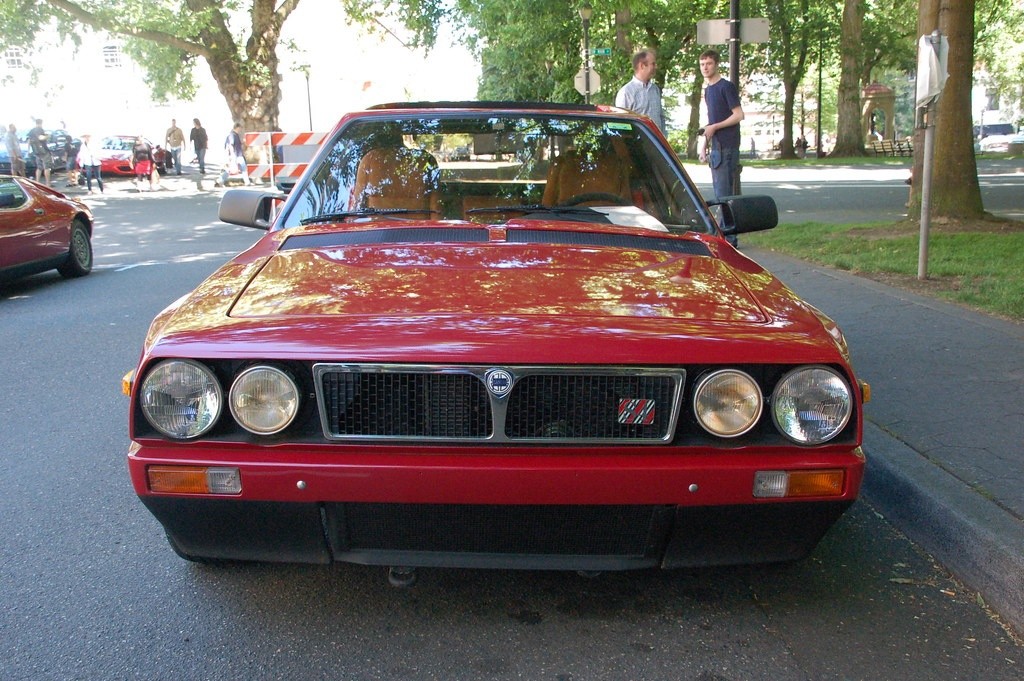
[978,133,1024,153]
[74,133,158,177]
[0,129,81,176]
[120,101,871,570]
[0,173,95,281]
[432,145,470,164]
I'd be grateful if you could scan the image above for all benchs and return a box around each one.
[436,180,544,223]
[871,139,914,158]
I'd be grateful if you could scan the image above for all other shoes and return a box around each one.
[88,191,92,195]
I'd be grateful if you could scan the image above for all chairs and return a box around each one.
[542,147,635,209]
[350,146,441,224]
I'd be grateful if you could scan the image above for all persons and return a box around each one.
[5,119,105,195]
[796,137,808,154]
[164,119,186,174]
[188,117,208,173]
[751,137,755,159]
[216,123,256,186]
[699,48,744,249]
[616,50,668,142]
[130,135,156,192]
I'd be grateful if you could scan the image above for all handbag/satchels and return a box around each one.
[78,168,85,185]
